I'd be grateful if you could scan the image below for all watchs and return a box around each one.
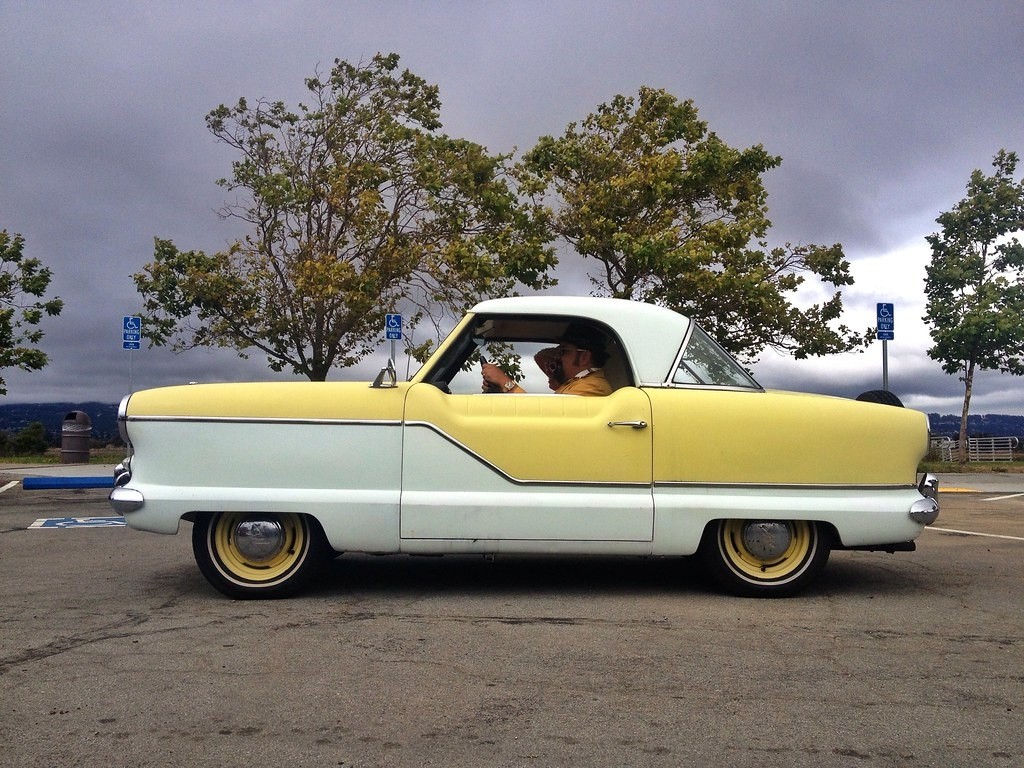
[502,380,516,393]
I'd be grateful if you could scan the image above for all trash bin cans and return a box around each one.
[61,411,91,463]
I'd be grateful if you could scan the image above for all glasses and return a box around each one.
[543,361,562,373]
[554,345,587,355]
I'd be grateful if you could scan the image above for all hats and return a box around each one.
[556,322,613,362]
[535,346,561,377]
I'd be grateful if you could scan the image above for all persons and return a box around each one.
[481,322,613,396]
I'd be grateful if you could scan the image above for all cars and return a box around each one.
[105,296,942,599]
